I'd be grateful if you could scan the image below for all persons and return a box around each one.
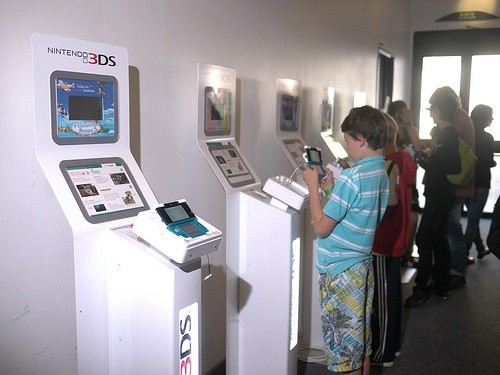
[303,105,390,375]
[370,112,414,367]
[464,105,497,265]
[388,86,476,308]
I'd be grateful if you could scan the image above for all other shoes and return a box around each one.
[404,286,431,308]
[478,250,490,259]
[430,285,448,300]
[465,256,474,264]
[370,357,394,367]
[450,275,459,289]
[394,348,401,357]
[458,276,465,288]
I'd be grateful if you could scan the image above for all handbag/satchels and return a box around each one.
[444,138,478,186]
